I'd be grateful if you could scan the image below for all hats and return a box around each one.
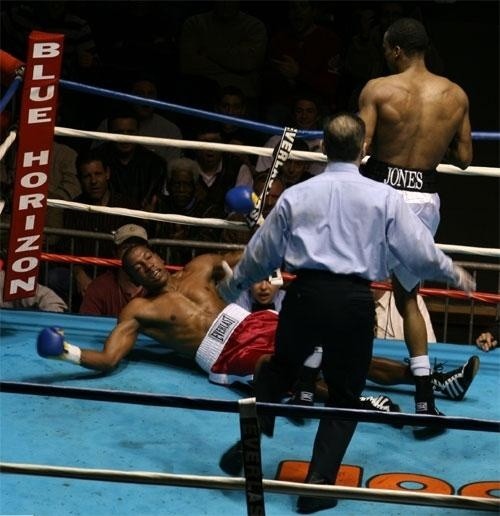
[114,224,148,245]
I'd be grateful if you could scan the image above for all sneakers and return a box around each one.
[219,445,243,477]
[297,496,337,514]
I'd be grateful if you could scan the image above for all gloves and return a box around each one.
[37,326,81,365]
[227,185,265,233]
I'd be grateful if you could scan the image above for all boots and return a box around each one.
[284,364,320,406]
[359,395,403,429]
[430,357,479,401]
[413,375,445,438]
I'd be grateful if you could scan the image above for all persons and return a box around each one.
[275,18,475,434]
[1,1,500,348]
[214,116,478,515]
[36,243,480,430]
